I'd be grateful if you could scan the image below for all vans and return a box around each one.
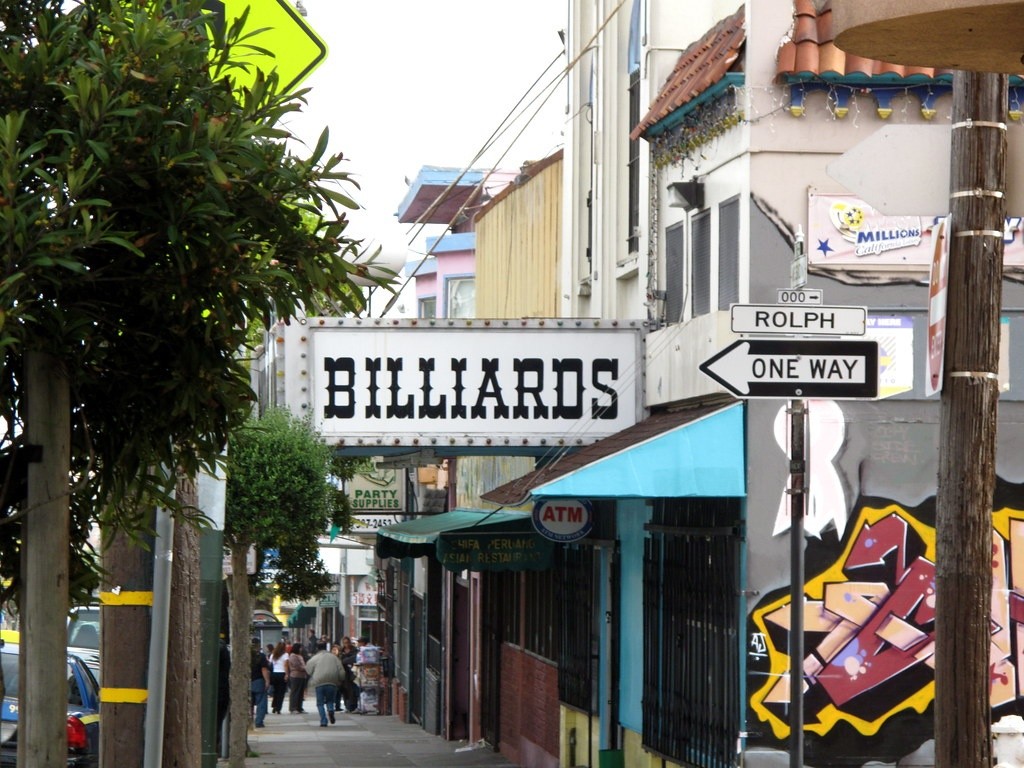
[66,607,100,695]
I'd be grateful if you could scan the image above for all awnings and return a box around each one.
[375,508,559,573]
[478,398,744,507]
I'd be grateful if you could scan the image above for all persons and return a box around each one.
[250,630,376,729]
[305,640,346,729]
[217,638,233,744]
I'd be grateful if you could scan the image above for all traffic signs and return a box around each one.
[699,339,879,402]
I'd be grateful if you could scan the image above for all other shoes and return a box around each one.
[273,708,281,715]
[346,709,353,713]
[351,708,361,714]
[336,707,344,711]
[321,723,327,727]
[256,723,265,728]
[330,718,335,724]
[292,708,304,714]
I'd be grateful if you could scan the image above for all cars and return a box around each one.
[1,643,100,767]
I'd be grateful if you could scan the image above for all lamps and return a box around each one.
[666,182,703,212]
